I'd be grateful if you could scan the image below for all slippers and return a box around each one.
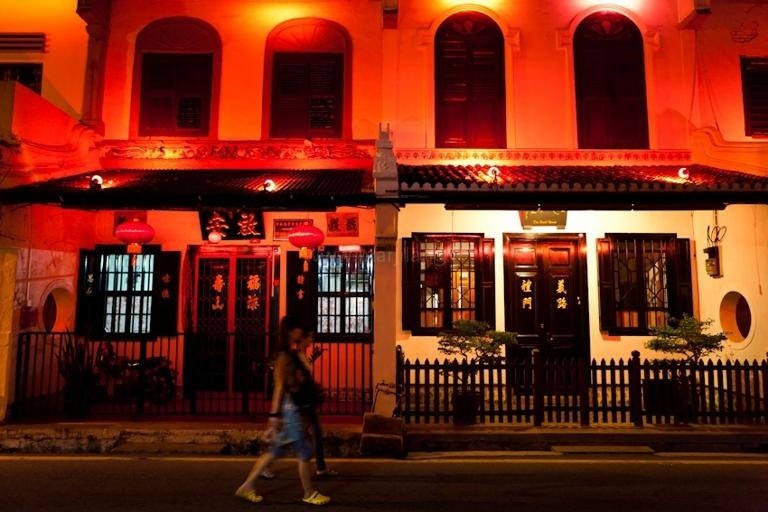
[235,486,264,503]
[302,490,331,506]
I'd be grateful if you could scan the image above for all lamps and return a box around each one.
[209,230,224,244]
[262,180,276,193]
[488,165,501,185]
[88,175,104,190]
[678,167,689,181]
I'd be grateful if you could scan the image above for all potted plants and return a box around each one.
[436,318,518,422]
[52,325,104,419]
[642,312,730,425]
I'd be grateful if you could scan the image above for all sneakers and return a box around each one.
[316,468,339,476]
[262,469,276,479]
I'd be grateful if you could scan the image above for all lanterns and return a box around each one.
[286,220,325,273]
[115,216,155,264]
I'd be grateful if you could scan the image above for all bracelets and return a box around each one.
[266,411,282,417]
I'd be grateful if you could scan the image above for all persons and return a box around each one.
[255,321,338,478]
[232,310,332,505]
